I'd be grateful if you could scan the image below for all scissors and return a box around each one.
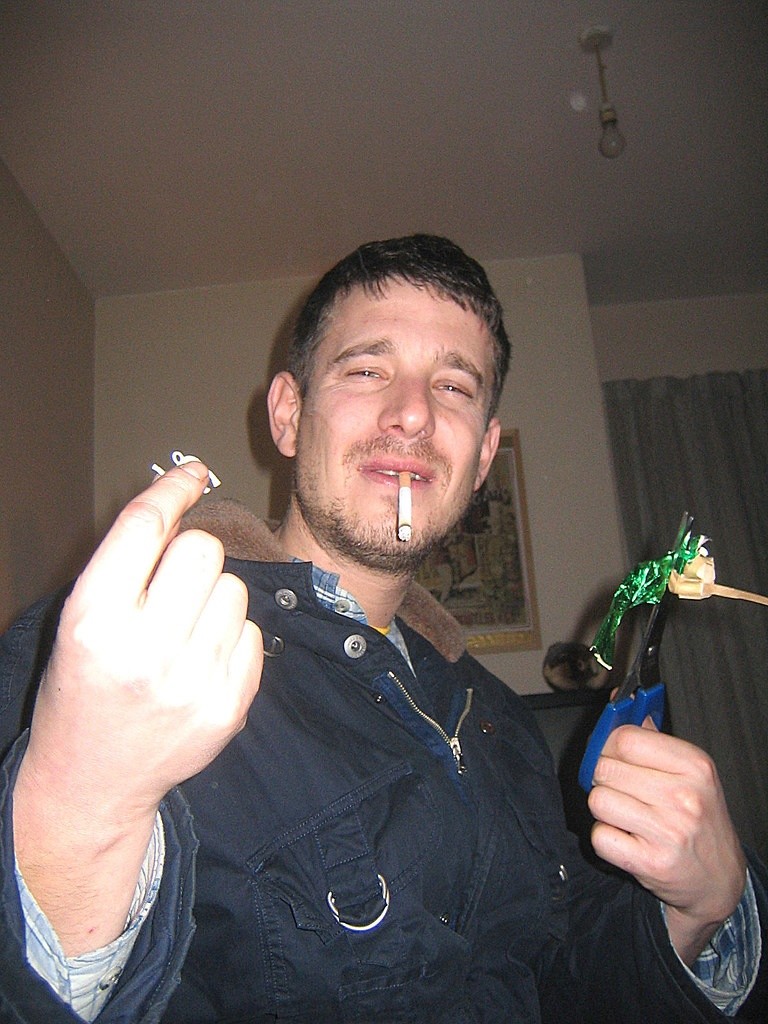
[576,511,697,794]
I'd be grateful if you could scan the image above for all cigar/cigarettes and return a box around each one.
[396,472,412,541]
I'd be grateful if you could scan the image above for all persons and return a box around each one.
[0,233,768,1024]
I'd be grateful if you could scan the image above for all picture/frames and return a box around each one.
[413,430,544,660]
